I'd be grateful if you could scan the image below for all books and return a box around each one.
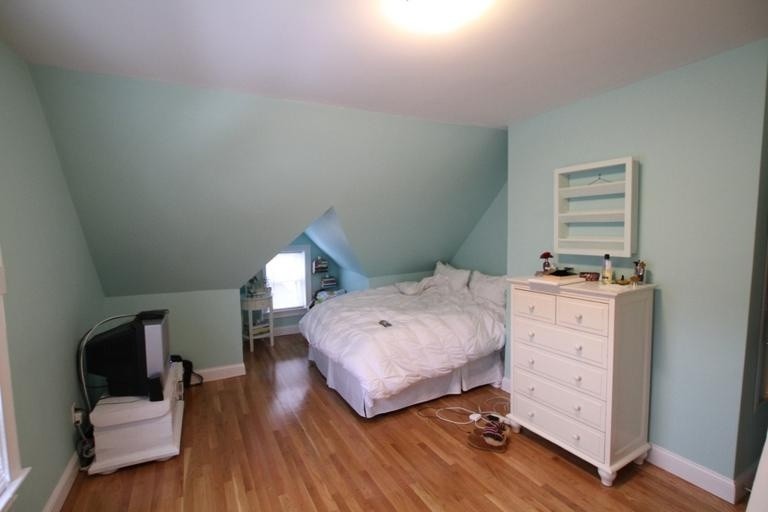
[322,277,335,287]
[528,277,586,286]
[314,261,328,273]
[541,270,579,281]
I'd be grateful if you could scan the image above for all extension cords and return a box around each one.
[75,413,82,425]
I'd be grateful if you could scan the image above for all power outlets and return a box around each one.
[72,402,78,423]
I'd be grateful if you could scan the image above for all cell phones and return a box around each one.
[379,320,392,327]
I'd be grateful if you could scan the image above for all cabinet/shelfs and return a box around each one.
[552,157,640,259]
[507,274,657,488]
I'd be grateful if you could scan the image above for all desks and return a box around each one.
[87,362,185,475]
[241,292,274,352]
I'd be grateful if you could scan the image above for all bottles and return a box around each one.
[600,253,612,288]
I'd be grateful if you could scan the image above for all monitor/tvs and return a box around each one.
[85,309,170,396]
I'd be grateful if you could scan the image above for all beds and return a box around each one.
[298,259,507,418]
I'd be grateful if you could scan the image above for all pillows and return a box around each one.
[469,270,507,306]
[433,260,471,288]
